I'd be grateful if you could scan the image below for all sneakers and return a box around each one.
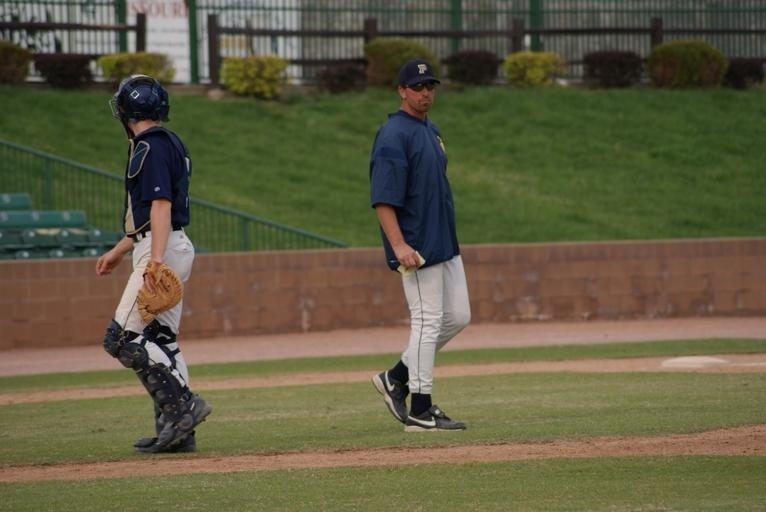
[159,394,212,448]
[134,430,197,454]
[370,370,408,423]
[404,405,466,432]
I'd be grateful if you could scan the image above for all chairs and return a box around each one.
[2,191,212,259]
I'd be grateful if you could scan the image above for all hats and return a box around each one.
[397,59,441,86]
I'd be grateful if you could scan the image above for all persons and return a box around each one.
[366,58,472,433]
[94,72,212,452]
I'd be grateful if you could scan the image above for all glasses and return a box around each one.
[408,82,435,92]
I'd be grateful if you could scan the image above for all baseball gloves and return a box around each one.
[137,262,182,325]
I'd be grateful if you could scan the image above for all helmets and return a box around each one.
[109,74,171,122]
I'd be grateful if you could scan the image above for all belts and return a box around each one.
[132,224,183,242]
